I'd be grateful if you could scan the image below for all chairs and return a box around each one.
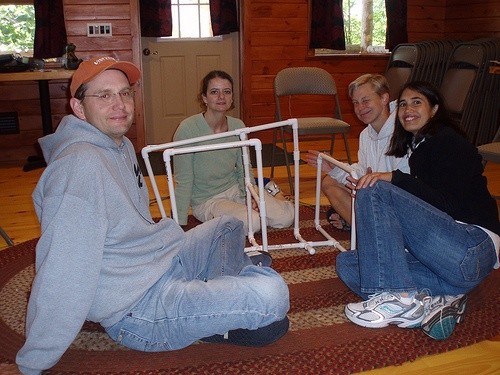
[271,66,353,196]
[383,32,500,167]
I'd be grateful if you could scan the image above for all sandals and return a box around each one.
[327,208,350,231]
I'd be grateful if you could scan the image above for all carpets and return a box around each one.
[138,143,307,177]
[0,206,500,375]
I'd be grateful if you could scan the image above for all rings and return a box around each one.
[372,174,375,178]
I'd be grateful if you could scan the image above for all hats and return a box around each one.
[70,56,141,98]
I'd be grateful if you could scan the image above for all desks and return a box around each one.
[0,69,77,172]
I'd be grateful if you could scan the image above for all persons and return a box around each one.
[305,73,412,231]
[336,80,500,340]
[173,70,294,236]
[0,57,290,375]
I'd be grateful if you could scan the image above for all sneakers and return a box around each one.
[245,250,273,267]
[200,316,290,346]
[345,292,430,328]
[420,294,467,340]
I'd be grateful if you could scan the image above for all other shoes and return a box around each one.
[264,181,280,196]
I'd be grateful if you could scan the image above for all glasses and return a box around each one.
[80,88,136,104]
